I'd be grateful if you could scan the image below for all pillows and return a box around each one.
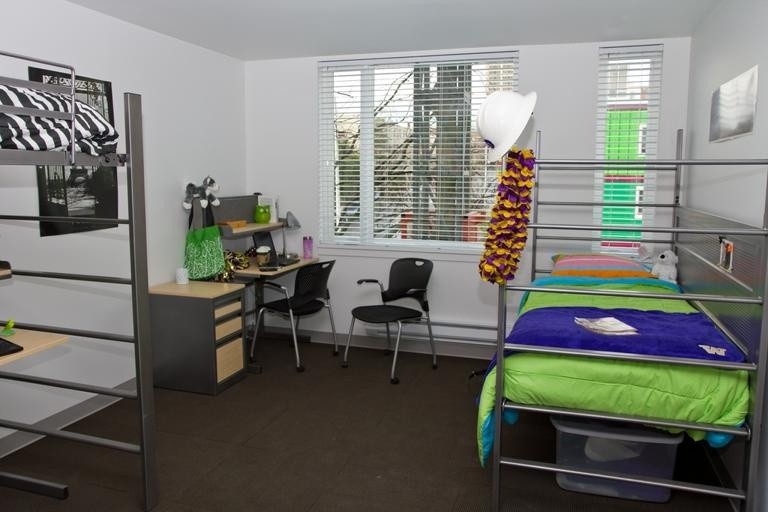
[545,250,656,281]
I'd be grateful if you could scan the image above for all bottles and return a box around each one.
[303,236,313,259]
[255,204,271,224]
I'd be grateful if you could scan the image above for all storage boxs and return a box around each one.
[545,413,686,503]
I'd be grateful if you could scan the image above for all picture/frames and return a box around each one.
[27,65,119,236]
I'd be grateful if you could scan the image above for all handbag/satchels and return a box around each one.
[183,201,226,281]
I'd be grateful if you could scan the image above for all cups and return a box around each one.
[257,245,271,267]
[271,207,278,223]
[176,268,192,285]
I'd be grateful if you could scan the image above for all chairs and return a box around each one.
[250,257,437,386]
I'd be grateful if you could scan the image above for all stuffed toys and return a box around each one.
[649,249,678,285]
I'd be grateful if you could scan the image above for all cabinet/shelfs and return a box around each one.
[148,280,248,396]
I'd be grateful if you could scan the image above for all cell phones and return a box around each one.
[260,267,278,272]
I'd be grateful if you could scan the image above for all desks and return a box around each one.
[224,249,319,346]
[1,326,72,499]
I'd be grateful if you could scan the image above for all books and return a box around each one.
[573,315,639,333]
[574,319,641,337]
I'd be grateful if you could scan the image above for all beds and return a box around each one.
[489,131,767,510]
[0,51,160,511]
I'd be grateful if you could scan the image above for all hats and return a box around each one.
[473,89,537,163]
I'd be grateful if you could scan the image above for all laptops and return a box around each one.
[252,230,300,266]
